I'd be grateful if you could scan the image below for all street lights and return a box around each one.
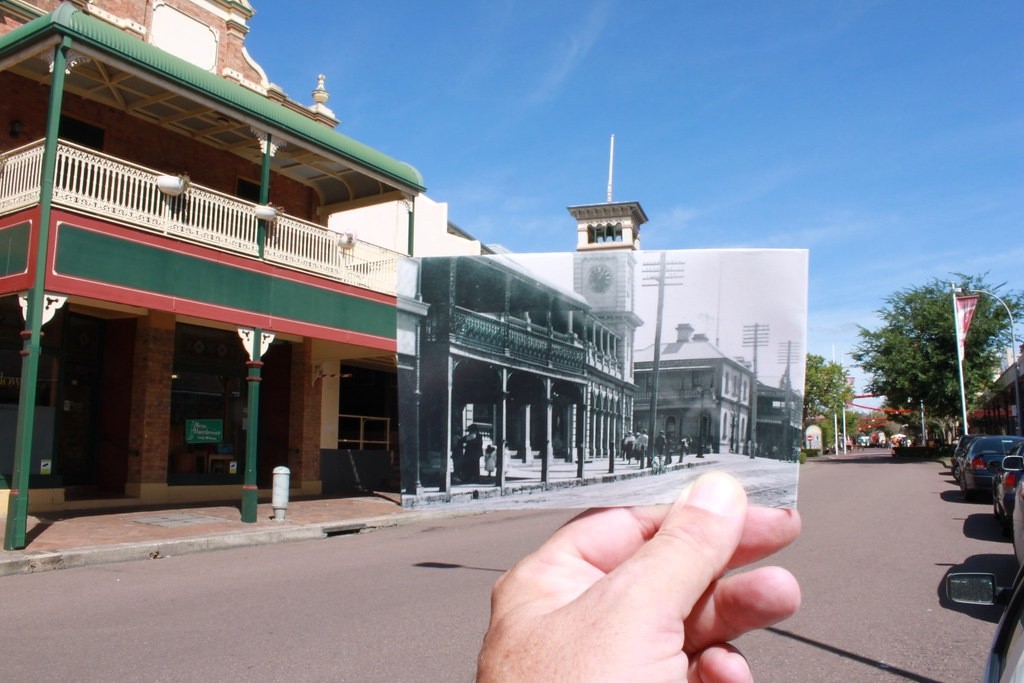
[953,287,1023,435]
[696,383,716,458]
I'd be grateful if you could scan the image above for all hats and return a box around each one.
[628,431,634,435]
[635,432,641,436]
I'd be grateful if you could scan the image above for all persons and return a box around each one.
[900,439,904,447]
[485,445,496,476]
[622,429,675,464]
[478,472,801,683]
[684,434,692,455]
[791,445,798,463]
[452,424,483,484]
[906,437,912,447]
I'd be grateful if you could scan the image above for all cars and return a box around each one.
[945,435,1024,683]
